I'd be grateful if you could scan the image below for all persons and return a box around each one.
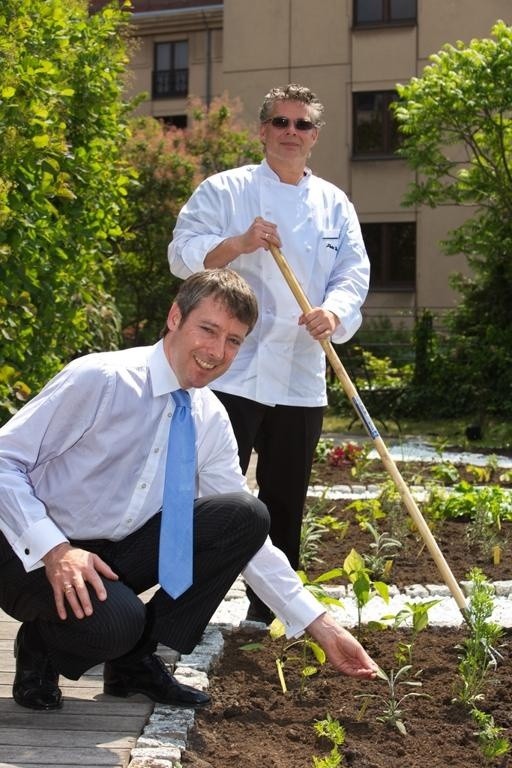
[165,82,372,629]
[0,266,378,711]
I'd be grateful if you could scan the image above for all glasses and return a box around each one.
[264,117,319,130]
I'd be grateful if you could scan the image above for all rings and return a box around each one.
[264,233,268,239]
[62,584,72,592]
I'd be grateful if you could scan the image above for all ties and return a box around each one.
[156,384,198,603]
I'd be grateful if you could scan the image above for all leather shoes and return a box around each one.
[11,623,65,711]
[102,652,212,709]
[247,601,275,625]
